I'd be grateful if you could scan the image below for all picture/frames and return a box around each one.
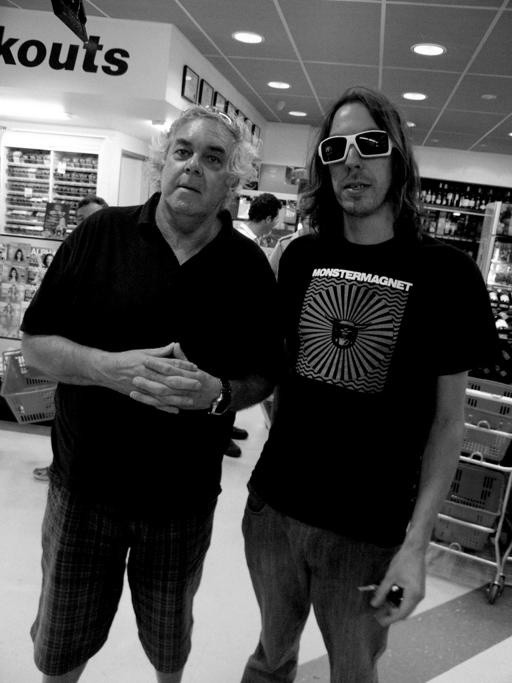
[181,64,261,147]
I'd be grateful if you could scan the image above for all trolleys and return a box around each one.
[408,376,512,605]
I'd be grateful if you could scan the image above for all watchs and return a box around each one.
[203,377,234,417]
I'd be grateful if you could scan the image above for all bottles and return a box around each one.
[417,182,512,242]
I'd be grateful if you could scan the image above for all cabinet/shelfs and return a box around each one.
[2,144,99,240]
[415,173,512,385]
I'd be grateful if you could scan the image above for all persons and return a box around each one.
[16,103,284,682]
[262,202,320,432]
[75,194,110,226]
[0,240,55,337]
[33,464,52,481]
[223,189,282,458]
[236,83,502,681]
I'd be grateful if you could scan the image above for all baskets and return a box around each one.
[0,348,57,425]
[434,462,505,550]
[462,376,512,461]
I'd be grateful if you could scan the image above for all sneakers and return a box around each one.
[33,467,49,480]
[225,426,248,457]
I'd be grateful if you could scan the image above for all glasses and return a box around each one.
[317,130,396,165]
